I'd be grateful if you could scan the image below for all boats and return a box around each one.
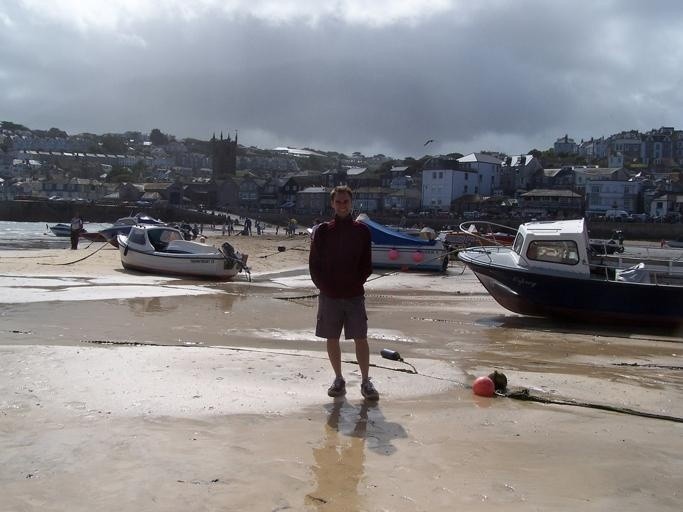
[98,213,168,247]
[382,224,421,235]
[665,240,683,247]
[434,220,625,258]
[99,217,169,242]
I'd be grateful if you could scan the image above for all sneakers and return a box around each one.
[361,383,380,400]
[328,380,346,396]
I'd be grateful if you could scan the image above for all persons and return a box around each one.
[71,211,83,250]
[309,186,380,402]
